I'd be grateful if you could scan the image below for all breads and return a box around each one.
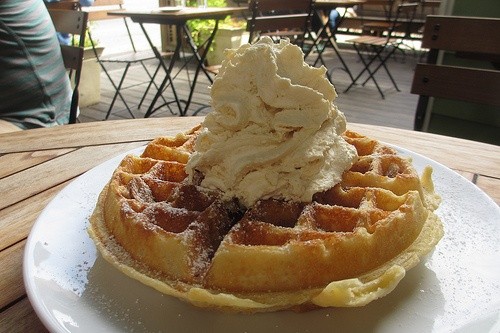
[85,122,447,311]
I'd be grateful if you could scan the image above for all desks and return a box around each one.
[103,6,251,116]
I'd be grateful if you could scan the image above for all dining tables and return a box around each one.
[0,116,499,332]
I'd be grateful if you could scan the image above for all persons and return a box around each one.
[0,0,93,134]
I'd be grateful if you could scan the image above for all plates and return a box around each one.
[159,6,183,12]
[21,134,500,333]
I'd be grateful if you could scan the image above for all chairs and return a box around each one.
[46,8,89,124]
[236,0,442,100]
[73,0,175,119]
[409,13,500,145]
